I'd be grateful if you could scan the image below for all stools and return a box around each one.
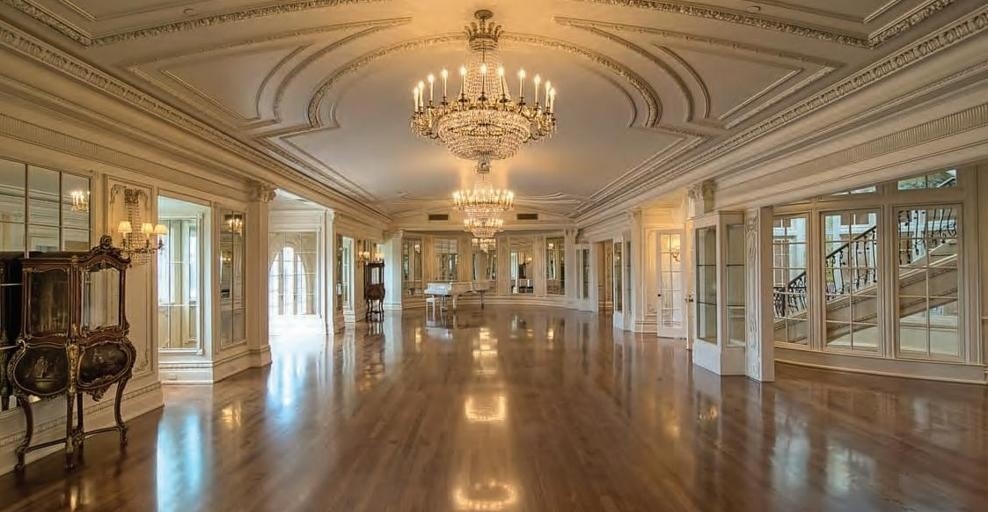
[426,296,441,311]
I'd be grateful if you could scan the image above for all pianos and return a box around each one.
[424,282,490,328]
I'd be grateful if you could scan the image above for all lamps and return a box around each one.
[112,186,166,254]
[411,11,559,175]
